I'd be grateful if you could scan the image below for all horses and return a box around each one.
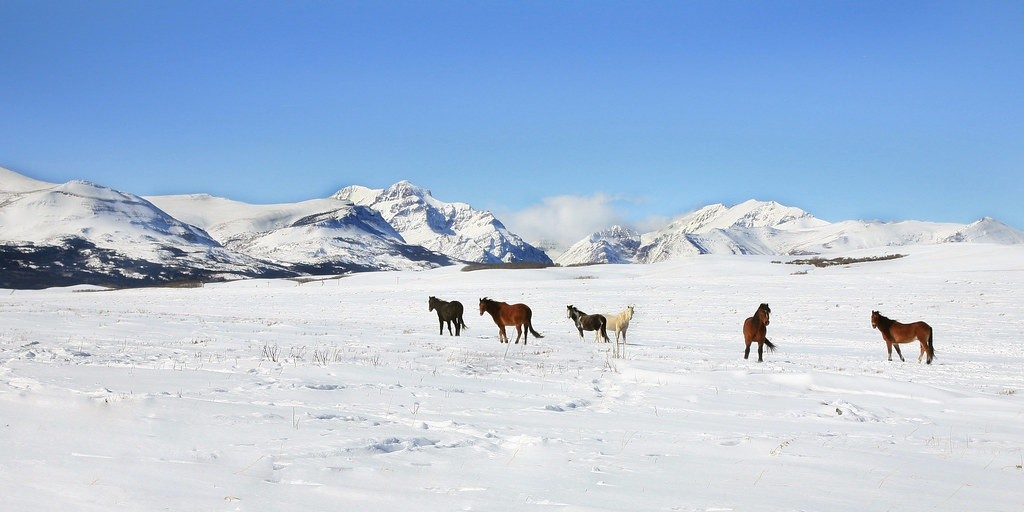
[594,306,634,346]
[566,305,611,344]
[479,297,545,346]
[871,310,938,364]
[428,295,469,336]
[743,303,778,363]
